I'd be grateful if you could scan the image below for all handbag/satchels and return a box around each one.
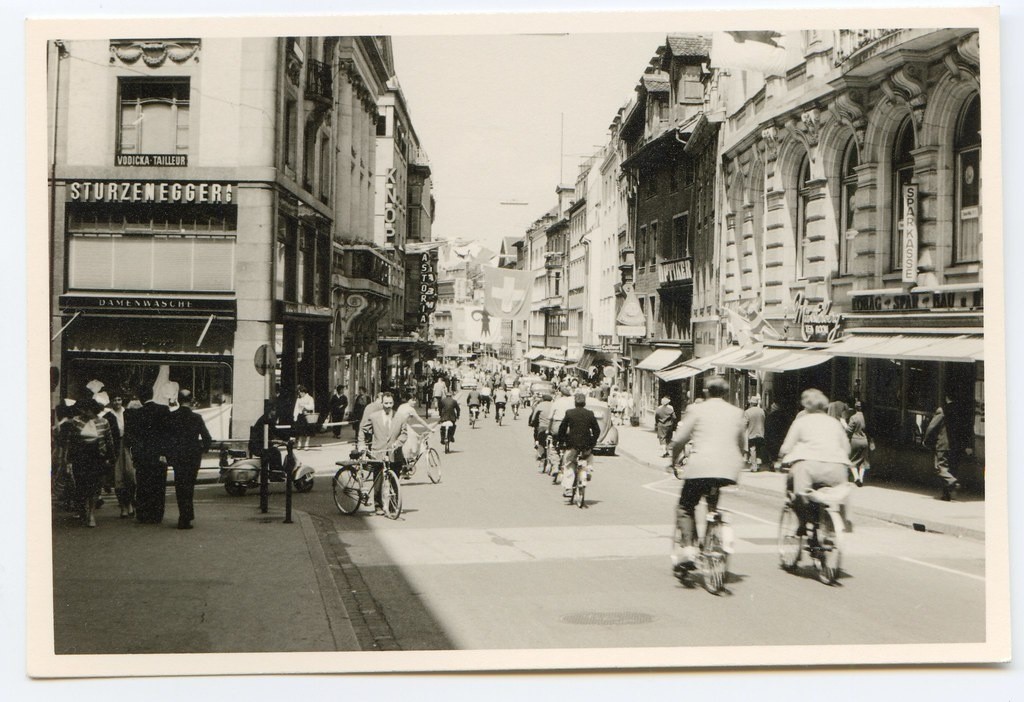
[865,434,876,450]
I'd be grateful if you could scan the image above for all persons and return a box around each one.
[292,387,316,450]
[357,393,408,515]
[922,392,974,500]
[327,364,630,481]
[776,389,852,553]
[671,376,747,572]
[555,394,601,497]
[248,403,288,482]
[52,386,213,530]
[655,392,873,488]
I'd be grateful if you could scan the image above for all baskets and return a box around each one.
[304,409,320,423]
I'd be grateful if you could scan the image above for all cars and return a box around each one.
[554,399,619,456]
[460,373,477,390]
[502,375,557,398]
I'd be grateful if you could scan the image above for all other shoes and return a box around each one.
[663,453,669,458]
[137,512,162,524]
[537,456,541,460]
[178,518,193,529]
[563,490,573,497]
[375,506,385,514]
[675,554,696,573]
[940,482,962,500]
[854,478,864,487]
[768,462,775,472]
[750,468,758,472]
[79,512,96,527]
[120,503,135,519]
[296,441,309,450]
[551,470,559,476]
[587,473,591,481]
[270,477,285,482]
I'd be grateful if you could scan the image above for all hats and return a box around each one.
[770,402,782,411]
[748,396,760,405]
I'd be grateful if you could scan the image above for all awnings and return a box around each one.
[635,335,984,383]
[520,347,598,372]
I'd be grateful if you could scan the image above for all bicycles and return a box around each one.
[522,396,528,408]
[665,456,732,594]
[482,402,489,420]
[443,420,451,454]
[498,408,504,426]
[512,402,518,420]
[534,433,588,508]
[331,446,404,520]
[772,461,844,584]
[400,430,441,485]
[470,405,478,429]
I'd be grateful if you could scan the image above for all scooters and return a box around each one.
[220,435,315,495]
